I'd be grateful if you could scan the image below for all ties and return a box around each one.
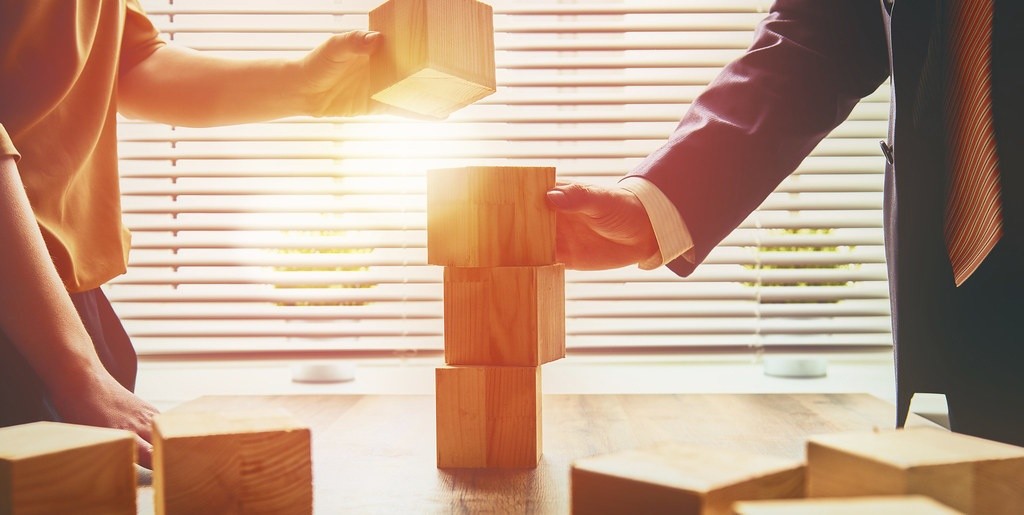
[946,0,1007,287]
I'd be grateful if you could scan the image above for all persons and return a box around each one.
[547,0,1024,450]
[2,0,454,480]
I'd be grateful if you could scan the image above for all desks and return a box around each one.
[136,390,948,514]
